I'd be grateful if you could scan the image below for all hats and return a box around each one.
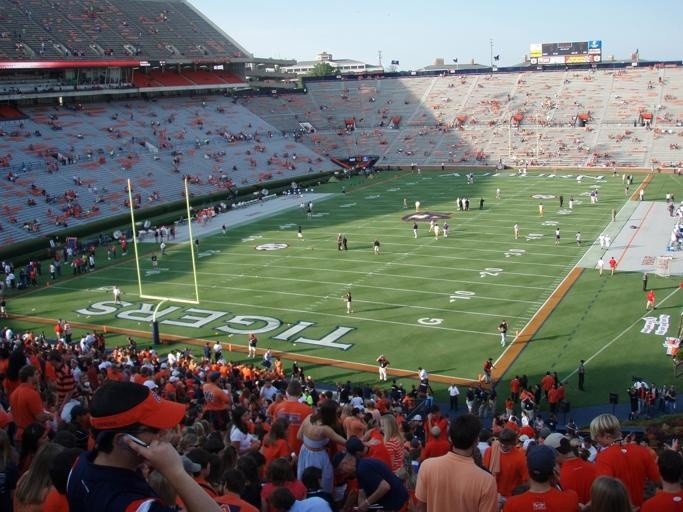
[161,363,180,382]
[87,380,187,431]
[143,379,158,390]
[210,372,220,380]
[181,455,202,474]
[411,414,423,421]
[526,443,557,477]
[332,452,346,469]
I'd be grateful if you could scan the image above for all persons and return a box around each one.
[0,0,682,511]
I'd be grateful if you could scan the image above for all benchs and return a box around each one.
[340,65,682,169]
[0,1,339,249]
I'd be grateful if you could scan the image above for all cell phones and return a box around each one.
[119,433,149,460]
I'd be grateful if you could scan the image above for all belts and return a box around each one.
[303,444,320,451]
